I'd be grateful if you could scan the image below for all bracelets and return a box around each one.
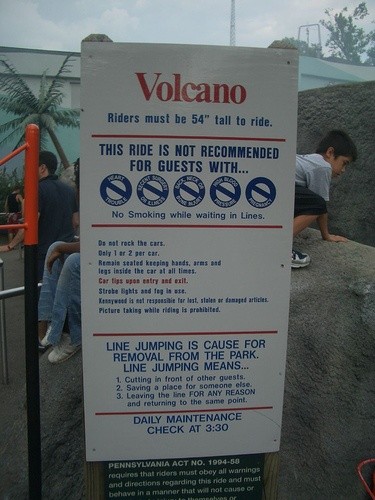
[7,245,12,251]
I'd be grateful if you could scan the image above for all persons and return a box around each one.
[292,130,357,268]
[4,185,23,250]
[0,150,73,324]
[38,243,82,364]
[36,238,69,353]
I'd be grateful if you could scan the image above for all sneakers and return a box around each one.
[48,337,82,364]
[40,323,55,346]
[291,249,311,268]
